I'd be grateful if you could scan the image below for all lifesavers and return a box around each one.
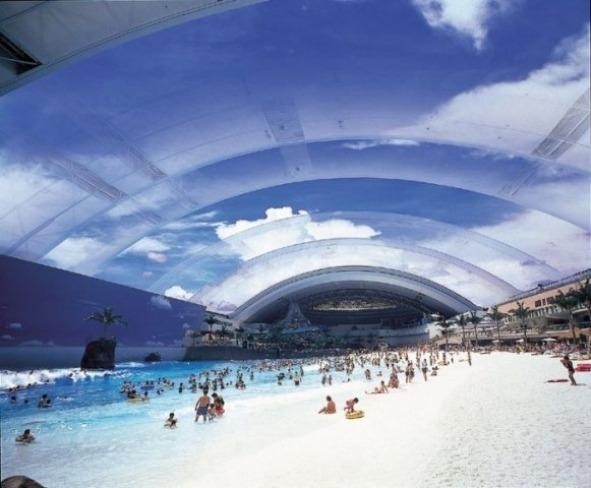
[128,397,143,403]
[345,410,364,419]
[139,384,154,390]
[213,408,225,413]
[15,433,35,442]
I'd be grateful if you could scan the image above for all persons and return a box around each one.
[343,395,358,414]
[561,356,576,385]
[469,340,590,356]
[207,404,216,422]
[121,338,465,400]
[213,396,224,416]
[195,387,210,422]
[165,412,177,423]
[165,420,178,430]
[8,384,50,408]
[15,426,35,443]
[318,395,336,414]
